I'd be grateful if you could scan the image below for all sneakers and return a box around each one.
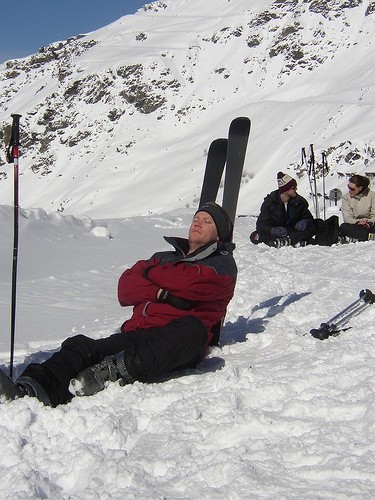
[0,368,24,398]
[75,361,118,395]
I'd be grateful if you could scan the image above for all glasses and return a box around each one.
[290,186,295,189]
[347,185,362,191]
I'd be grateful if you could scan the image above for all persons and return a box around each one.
[256,172,329,249]
[0,201,238,408]
[339,175,375,243]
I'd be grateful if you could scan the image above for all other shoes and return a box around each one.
[368,233,375,240]
[341,237,353,244]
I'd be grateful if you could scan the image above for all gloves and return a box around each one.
[143,265,152,278]
[271,227,288,237]
[294,218,310,231]
[159,290,192,309]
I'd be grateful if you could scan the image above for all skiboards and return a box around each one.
[198,115,251,346]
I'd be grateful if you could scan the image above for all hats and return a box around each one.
[194,202,232,242]
[276,172,296,192]
